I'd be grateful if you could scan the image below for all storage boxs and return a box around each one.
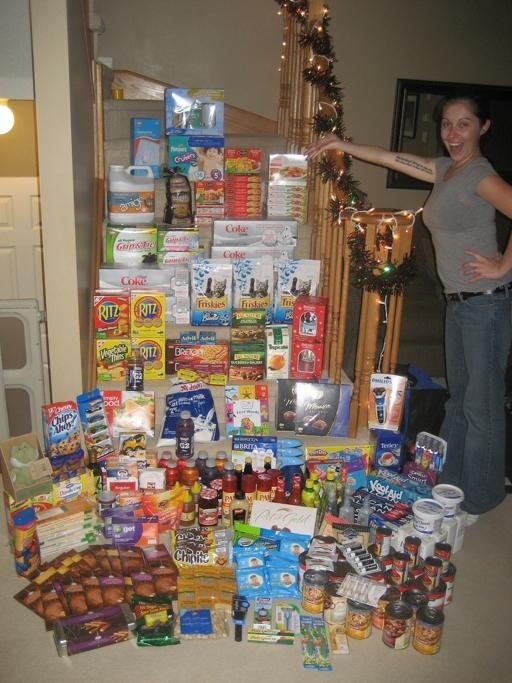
[98,219,299,327]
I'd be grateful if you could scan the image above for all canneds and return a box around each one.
[298,526,457,656]
[197,489,218,527]
[96,492,117,517]
[125,357,144,391]
[210,479,223,519]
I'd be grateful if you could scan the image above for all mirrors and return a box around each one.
[385,79,511,190]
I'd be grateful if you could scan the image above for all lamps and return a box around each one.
[0,98,16,133]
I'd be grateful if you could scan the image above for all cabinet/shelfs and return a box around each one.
[1,176,52,435]
[1,0,37,101]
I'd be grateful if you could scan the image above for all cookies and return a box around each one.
[198,345,227,361]
[144,360,163,370]
[134,317,161,328]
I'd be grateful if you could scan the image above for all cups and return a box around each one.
[110,87,124,100]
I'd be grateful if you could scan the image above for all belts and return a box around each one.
[448,282,512,300]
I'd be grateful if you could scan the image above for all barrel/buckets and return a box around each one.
[107,164,155,224]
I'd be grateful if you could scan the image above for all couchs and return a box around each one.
[342,205,447,383]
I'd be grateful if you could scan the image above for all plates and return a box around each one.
[276,435,304,467]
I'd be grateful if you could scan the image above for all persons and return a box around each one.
[304,86,512,526]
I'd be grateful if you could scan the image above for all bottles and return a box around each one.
[160,450,275,526]
[266,463,369,524]
[228,417,271,436]
[126,343,145,391]
[177,410,193,458]
[13,507,39,575]
[413,443,441,470]
